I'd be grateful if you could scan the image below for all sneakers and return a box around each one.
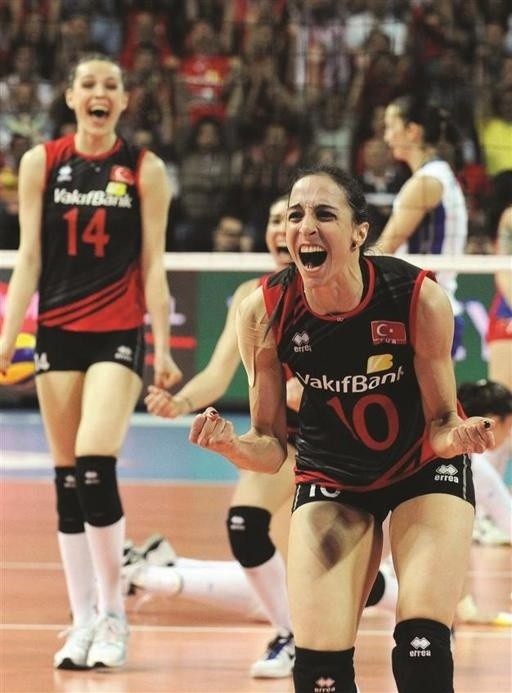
[54,625,88,670]
[88,613,130,668]
[249,633,294,677]
[121,541,141,594]
[142,535,177,566]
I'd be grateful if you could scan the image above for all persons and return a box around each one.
[144,196,398,679]
[189,169,494,692]
[122,378,512,627]
[367,94,512,535]
[471,209,512,548]
[1,0,512,253]
[1,53,180,669]
